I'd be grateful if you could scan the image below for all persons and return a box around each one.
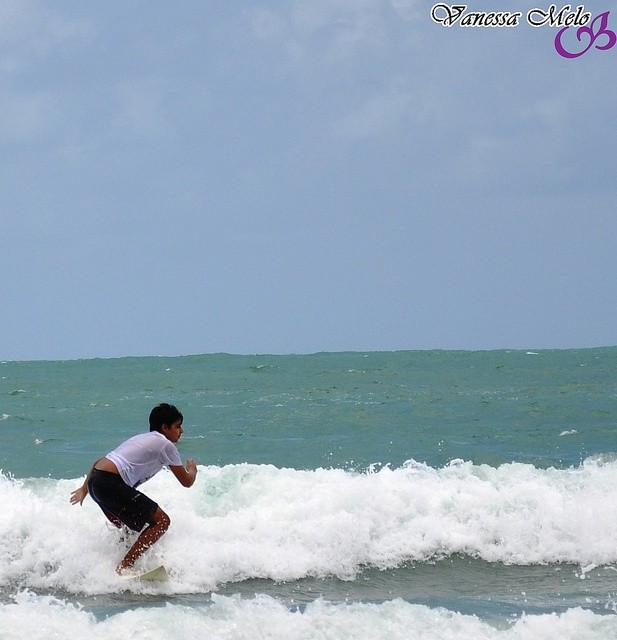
[70,403,198,575]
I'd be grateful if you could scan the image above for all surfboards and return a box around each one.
[122,565,169,582]
